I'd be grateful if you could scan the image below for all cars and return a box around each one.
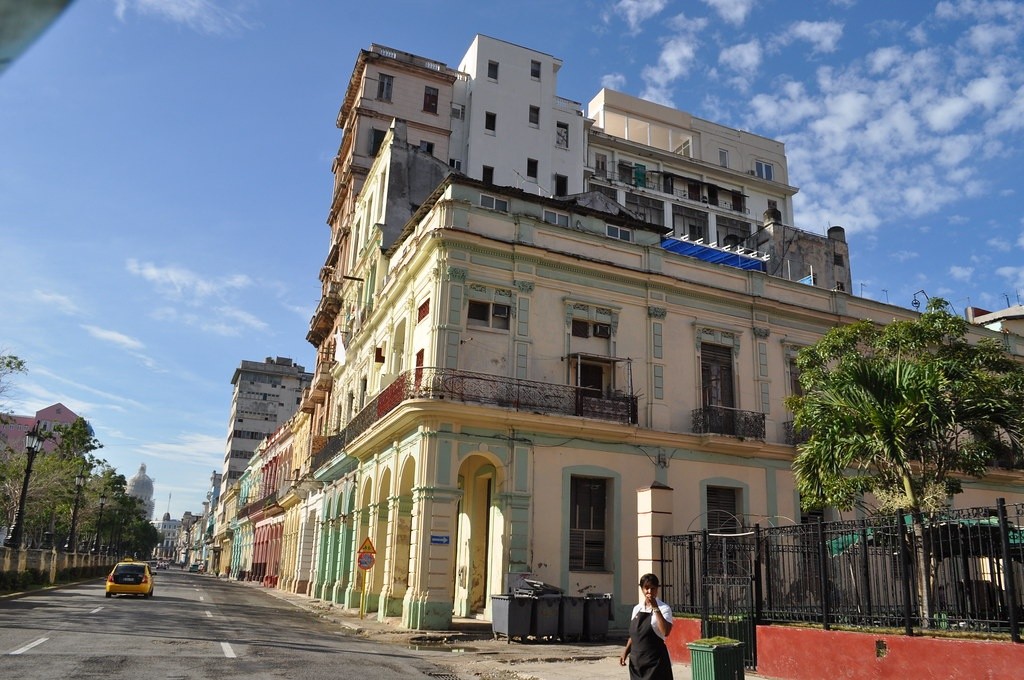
[105,562,157,597]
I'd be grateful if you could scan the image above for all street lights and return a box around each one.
[3,420,47,548]
[64,467,85,552]
[91,494,106,555]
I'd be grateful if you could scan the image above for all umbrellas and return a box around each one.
[819,514,1024,560]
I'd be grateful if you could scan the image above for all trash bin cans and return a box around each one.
[557,593,585,642]
[488,594,533,644]
[513,578,566,641]
[583,593,616,641]
[684,636,746,680]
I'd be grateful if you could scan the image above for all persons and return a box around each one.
[618,573,675,680]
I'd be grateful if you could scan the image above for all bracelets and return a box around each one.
[653,606,660,612]
